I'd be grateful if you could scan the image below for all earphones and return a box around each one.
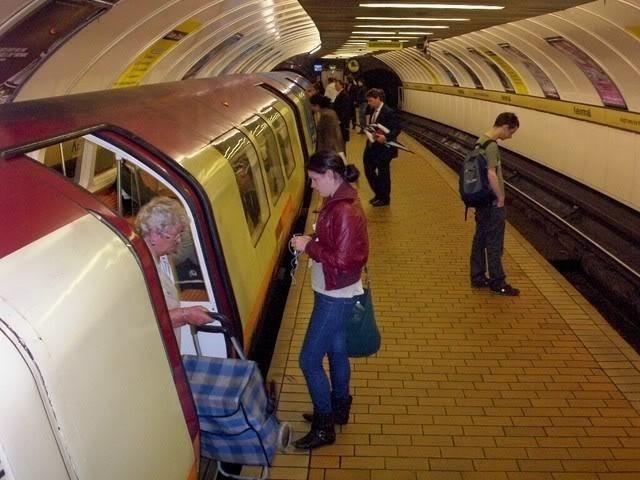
[295,259,298,264]
[294,250,297,255]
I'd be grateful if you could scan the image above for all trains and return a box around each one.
[0,71,321,480]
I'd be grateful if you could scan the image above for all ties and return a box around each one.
[373,110,378,123]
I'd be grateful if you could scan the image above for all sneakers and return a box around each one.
[472,274,519,295]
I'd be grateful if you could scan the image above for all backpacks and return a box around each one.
[460,143,496,207]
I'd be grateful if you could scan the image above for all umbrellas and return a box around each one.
[352,123,416,154]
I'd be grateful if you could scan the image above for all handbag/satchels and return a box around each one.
[341,129,350,141]
[182,354,277,466]
[346,288,381,357]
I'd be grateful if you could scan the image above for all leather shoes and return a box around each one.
[370,198,389,206]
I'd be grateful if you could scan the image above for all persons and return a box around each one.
[112,159,144,217]
[459,112,520,296]
[362,88,401,207]
[134,197,215,354]
[290,152,369,450]
[305,75,368,153]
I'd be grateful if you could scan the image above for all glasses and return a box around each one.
[160,232,184,240]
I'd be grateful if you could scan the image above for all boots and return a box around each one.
[292,395,352,449]
